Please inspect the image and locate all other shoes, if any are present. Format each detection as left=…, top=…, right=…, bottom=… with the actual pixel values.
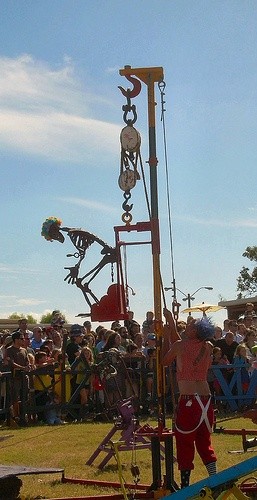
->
left=145, top=394, right=151, bottom=400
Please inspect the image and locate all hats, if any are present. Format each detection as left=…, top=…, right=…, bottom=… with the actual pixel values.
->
left=147, top=333, right=156, bottom=339
left=70, top=328, right=83, bottom=337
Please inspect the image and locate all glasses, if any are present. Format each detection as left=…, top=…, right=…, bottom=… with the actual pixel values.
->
left=18, top=338, right=25, bottom=340
left=25, top=338, right=30, bottom=340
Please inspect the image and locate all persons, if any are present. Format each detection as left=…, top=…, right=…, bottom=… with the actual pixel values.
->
left=0, top=303, right=257, bottom=426
left=160, top=308, right=218, bottom=489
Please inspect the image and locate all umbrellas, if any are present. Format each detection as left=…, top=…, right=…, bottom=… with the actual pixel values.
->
left=181, top=302, right=223, bottom=317
